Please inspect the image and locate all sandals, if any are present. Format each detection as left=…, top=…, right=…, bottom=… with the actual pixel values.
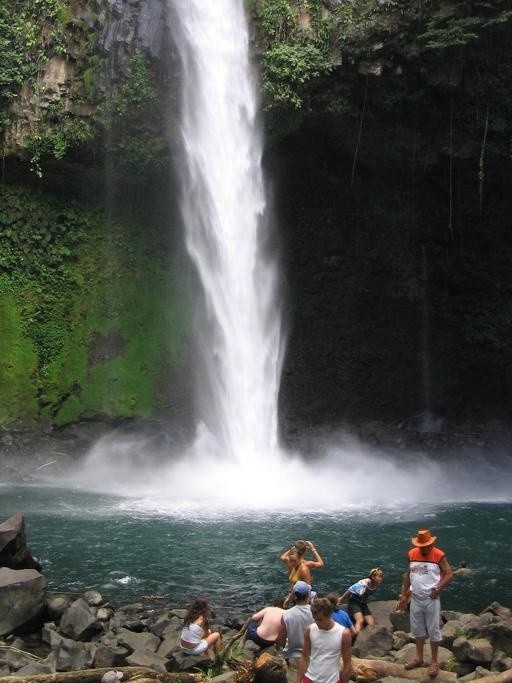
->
left=428, top=663, right=438, bottom=676
left=405, top=659, right=424, bottom=668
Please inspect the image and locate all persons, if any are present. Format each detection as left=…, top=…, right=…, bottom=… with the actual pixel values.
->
left=453, top=561, right=471, bottom=575
left=395, top=530, right=454, bottom=675
left=240, top=540, right=383, bottom=683
left=176, top=598, right=222, bottom=662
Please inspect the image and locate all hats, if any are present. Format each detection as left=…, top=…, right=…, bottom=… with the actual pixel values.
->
left=293, top=581, right=312, bottom=596
left=412, top=528, right=437, bottom=547
